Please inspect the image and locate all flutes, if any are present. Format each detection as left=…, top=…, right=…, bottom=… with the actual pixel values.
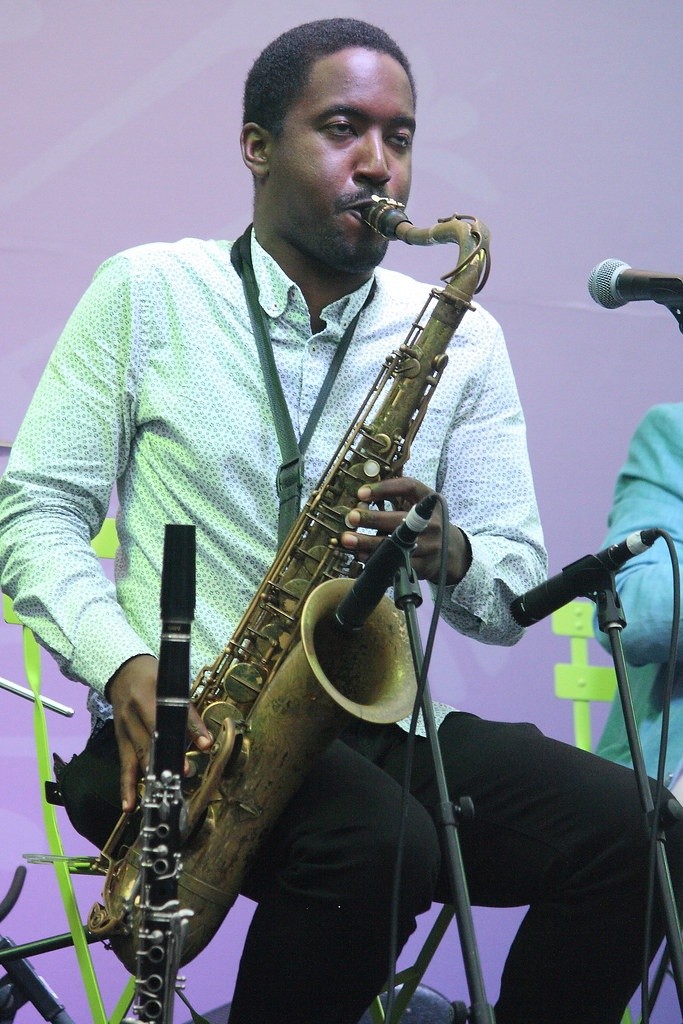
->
left=130, top=523, right=195, bottom=1024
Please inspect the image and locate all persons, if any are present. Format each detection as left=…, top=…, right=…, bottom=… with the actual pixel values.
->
left=2, top=19, right=683, bottom=1024
left=595, top=402, right=681, bottom=799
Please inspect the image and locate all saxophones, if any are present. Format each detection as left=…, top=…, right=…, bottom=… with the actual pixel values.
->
left=103, top=199, right=493, bottom=979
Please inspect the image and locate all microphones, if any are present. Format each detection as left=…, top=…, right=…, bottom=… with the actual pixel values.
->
left=509, top=528, right=663, bottom=627
left=334, top=493, right=439, bottom=632
left=587, top=259, right=683, bottom=309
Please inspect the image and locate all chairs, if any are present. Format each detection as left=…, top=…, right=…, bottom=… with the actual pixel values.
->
left=0, top=516, right=393, bottom=1024
left=548, top=599, right=621, bottom=752
